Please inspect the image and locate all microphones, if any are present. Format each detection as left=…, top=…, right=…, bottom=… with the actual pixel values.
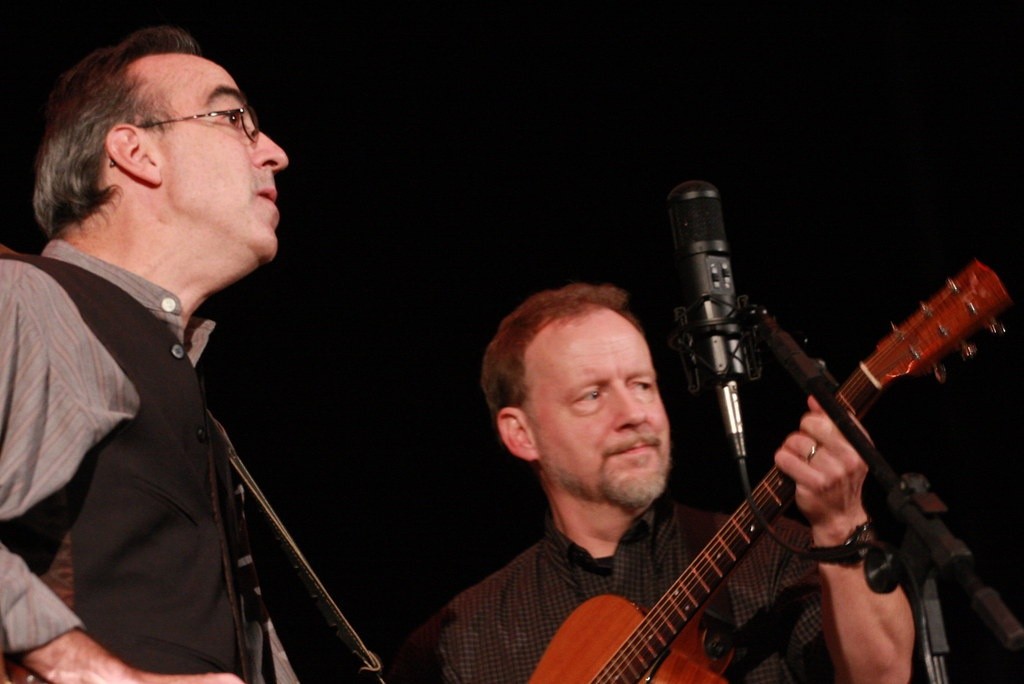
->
left=666, top=181, right=752, bottom=458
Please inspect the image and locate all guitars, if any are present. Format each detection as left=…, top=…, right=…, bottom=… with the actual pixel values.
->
left=521, top=257, right=1015, bottom=684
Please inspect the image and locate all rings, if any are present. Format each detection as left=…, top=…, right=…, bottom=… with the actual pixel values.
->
left=807, top=441, right=824, bottom=463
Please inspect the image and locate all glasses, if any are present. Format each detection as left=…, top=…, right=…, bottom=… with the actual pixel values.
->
left=109, top=102, right=261, bottom=168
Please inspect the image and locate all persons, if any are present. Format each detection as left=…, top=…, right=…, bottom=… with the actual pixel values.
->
left=388, top=282, right=914, bottom=684
left=1, top=27, right=300, bottom=683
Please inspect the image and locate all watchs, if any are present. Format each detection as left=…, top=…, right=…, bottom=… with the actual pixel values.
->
left=809, top=521, right=877, bottom=570
